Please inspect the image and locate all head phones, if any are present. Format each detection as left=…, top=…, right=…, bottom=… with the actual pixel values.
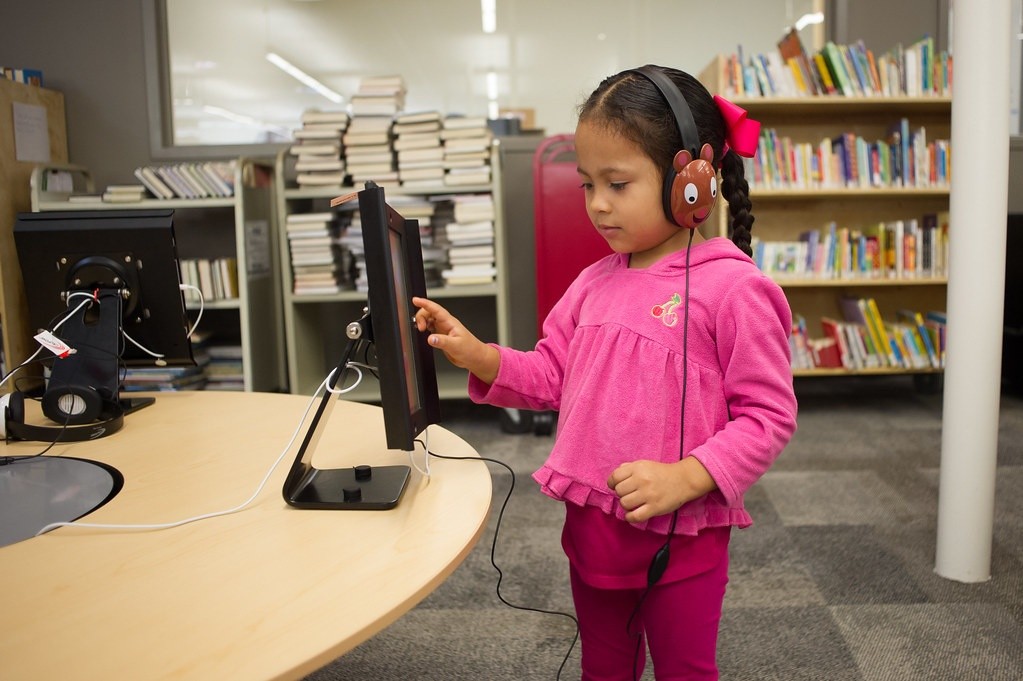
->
left=0, top=377, right=124, bottom=442
left=619, top=65, right=720, bottom=227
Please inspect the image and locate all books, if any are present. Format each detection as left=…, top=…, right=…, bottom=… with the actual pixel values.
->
left=0, top=38, right=953, bottom=393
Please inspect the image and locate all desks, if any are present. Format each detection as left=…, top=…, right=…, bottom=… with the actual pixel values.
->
left=0, top=392, right=493, bottom=680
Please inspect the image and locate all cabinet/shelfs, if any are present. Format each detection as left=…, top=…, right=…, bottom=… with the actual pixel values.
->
left=28, top=155, right=290, bottom=393
left=695, top=56, right=948, bottom=378
left=276, top=135, right=536, bottom=435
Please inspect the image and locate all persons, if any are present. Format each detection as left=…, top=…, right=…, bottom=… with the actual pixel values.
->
left=411, top=66, right=797, bottom=681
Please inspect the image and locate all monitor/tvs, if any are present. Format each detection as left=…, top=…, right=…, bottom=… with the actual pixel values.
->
left=356, top=189, right=441, bottom=450
left=14, top=208, right=198, bottom=368
